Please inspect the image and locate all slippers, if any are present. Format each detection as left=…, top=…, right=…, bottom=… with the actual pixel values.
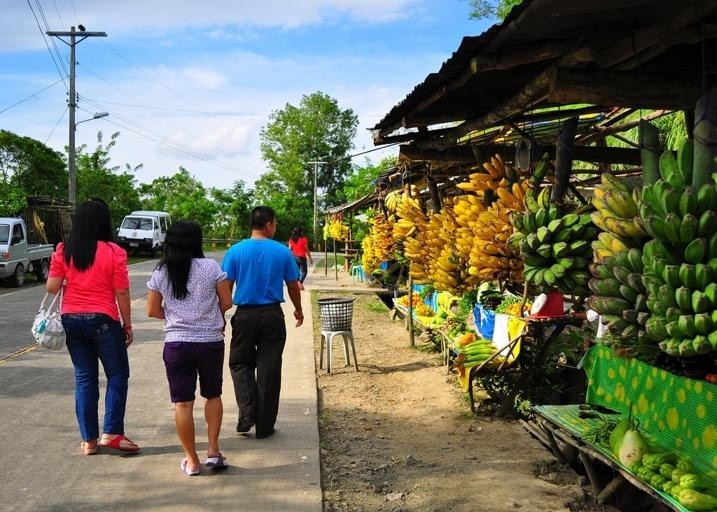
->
left=205, top=452, right=230, bottom=470
left=180, top=457, right=200, bottom=475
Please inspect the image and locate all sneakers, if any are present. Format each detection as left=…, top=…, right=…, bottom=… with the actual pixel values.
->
left=254, top=426, right=276, bottom=438
left=99, top=433, right=140, bottom=452
left=235, top=417, right=255, bottom=433
left=79, top=439, right=97, bottom=456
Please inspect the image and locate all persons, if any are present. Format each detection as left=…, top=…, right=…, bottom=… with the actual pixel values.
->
left=145, top=220, right=233, bottom=473
left=288, top=226, right=313, bottom=290
left=45, top=195, right=141, bottom=458
left=220, top=205, right=304, bottom=439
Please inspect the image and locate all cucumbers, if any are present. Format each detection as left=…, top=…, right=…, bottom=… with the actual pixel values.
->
left=630, top=452, right=717, bottom=512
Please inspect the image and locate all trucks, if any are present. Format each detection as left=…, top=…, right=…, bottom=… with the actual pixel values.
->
left=0, top=217, right=54, bottom=287
left=117, top=210, right=171, bottom=257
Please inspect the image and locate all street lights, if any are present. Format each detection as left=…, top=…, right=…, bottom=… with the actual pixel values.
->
left=75, top=112, right=109, bottom=131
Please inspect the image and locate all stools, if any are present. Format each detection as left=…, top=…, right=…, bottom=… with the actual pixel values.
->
left=318, top=331, right=359, bottom=375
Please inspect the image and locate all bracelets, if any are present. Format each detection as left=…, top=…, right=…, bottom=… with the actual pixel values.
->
left=122, top=323, right=133, bottom=331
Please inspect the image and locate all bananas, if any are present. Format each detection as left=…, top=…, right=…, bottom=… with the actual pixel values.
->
left=323, top=153, right=717, bottom=361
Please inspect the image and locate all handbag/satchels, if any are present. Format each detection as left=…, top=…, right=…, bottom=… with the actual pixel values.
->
left=30, top=307, right=67, bottom=351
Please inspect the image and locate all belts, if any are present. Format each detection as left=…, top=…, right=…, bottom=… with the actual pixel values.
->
left=62, top=312, right=106, bottom=322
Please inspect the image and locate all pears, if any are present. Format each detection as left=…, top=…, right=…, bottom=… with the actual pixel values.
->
left=608, top=403, right=648, bottom=468
